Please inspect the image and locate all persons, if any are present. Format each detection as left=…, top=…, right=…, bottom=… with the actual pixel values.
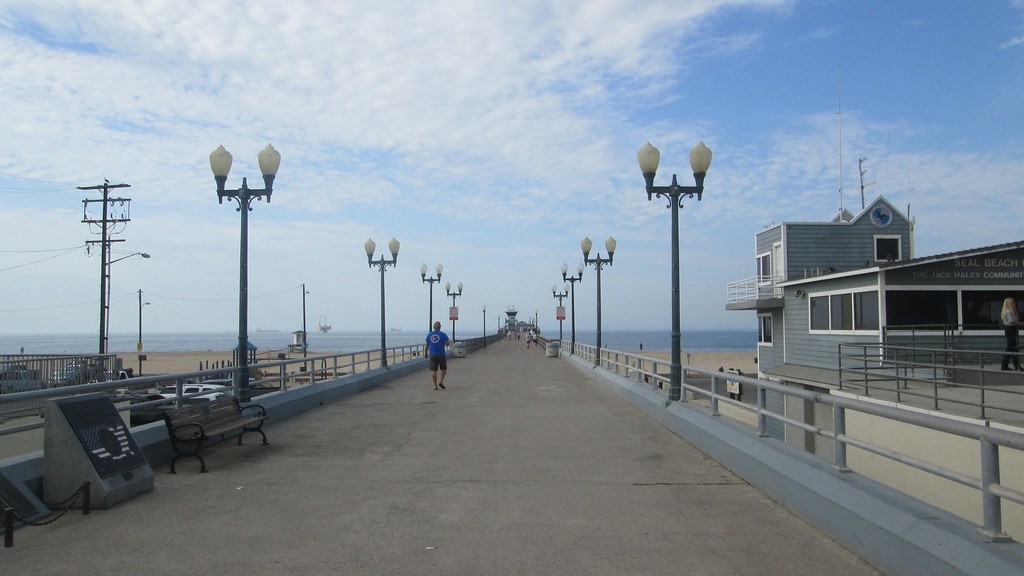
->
left=498, top=328, right=538, bottom=349
left=425, top=321, right=450, bottom=390
left=1001, top=298, right=1024, bottom=371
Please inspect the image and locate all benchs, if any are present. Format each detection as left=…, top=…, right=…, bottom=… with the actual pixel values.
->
left=160, top=395, right=269, bottom=474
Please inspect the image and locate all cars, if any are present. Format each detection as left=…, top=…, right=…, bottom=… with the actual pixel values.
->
left=108, top=376, right=252, bottom=408
left=1, top=357, right=98, bottom=397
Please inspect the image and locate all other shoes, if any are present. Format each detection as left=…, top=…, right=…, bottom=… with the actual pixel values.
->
left=434, top=387, right=438, bottom=390
left=439, top=382, right=446, bottom=389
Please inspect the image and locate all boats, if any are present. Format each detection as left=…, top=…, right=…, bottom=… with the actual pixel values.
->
left=316, top=323, right=331, bottom=331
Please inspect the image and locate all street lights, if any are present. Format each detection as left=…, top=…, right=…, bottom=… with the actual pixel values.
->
left=445, top=281, right=463, bottom=349
left=637, top=140, right=715, bottom=405
left=580, top=234, right=616, bottom=365
left=561, top=261, right=585, bottom=356
left=209, top=142, right=281, bottom=403
left=421, top=262, right=444, bottom=334
left=100, top=252, right=151, bottom=359
left=552, top=284, right=570, bottom=357
left=482, top=304, right=486, bottom=348
left=364, top=237, right=400, bottom=369
left=534, top=309, right=539, bottom=340
